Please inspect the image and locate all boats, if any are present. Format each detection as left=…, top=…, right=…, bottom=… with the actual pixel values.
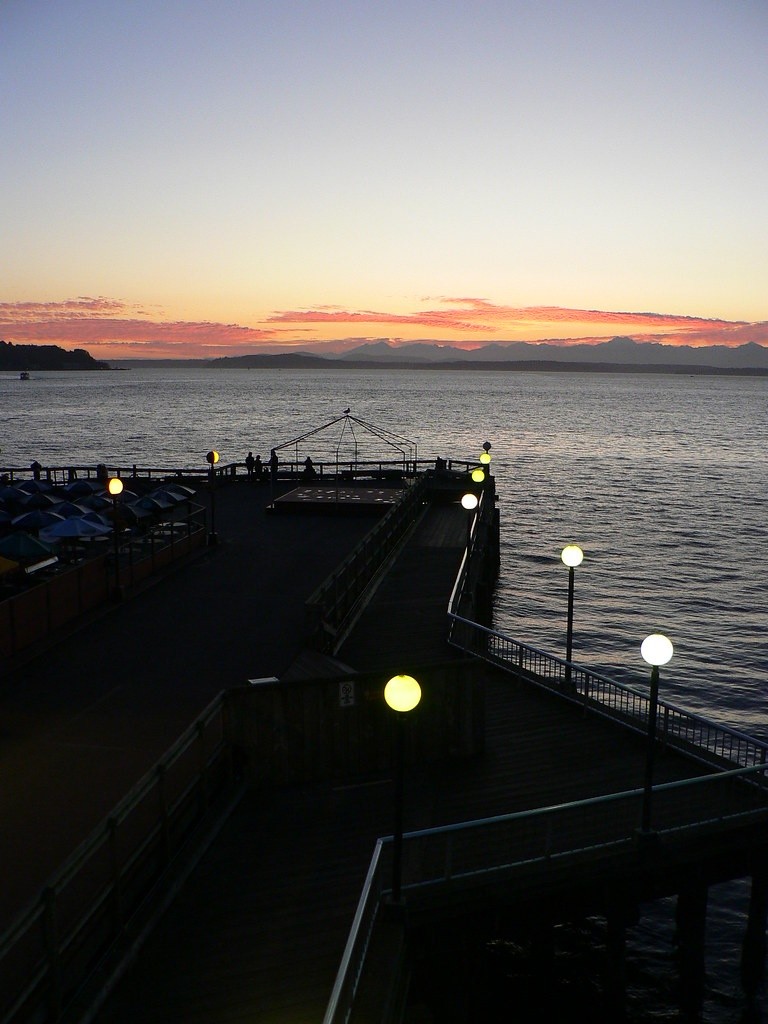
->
left=19, top=371, right=31, bottom=382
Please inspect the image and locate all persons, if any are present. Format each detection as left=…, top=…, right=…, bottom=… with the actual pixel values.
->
left=268, top=450, right=278, bottom=484
left=304, top=457, right=313, bottom=469
left=245, top=452, right=263, bottom=481
left=435, top=457, right=443, bottom=473
